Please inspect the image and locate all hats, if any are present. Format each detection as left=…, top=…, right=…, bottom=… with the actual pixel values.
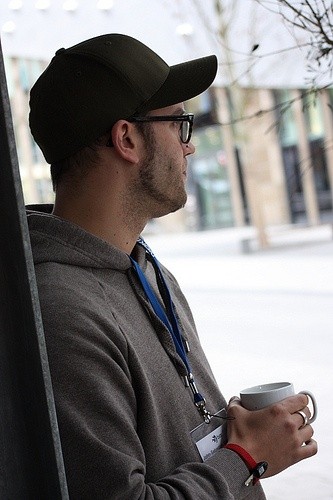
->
left=29, top=33, right=219, bottom=165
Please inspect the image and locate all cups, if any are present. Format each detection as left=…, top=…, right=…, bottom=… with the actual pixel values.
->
left=239, top=382, right=318, bottom=425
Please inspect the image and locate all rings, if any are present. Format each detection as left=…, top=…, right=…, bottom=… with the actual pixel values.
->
left=295, top=411, right=307, bottom=428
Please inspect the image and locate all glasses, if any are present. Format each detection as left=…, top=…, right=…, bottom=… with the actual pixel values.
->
left=130, top=112, right=194, bottom=143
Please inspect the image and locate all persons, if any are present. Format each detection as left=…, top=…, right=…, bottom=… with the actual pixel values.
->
left=23, top=33, right=317, bottom=500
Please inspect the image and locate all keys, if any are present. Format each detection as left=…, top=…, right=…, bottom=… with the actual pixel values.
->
left=244, top=462, right=268, bottom=486
